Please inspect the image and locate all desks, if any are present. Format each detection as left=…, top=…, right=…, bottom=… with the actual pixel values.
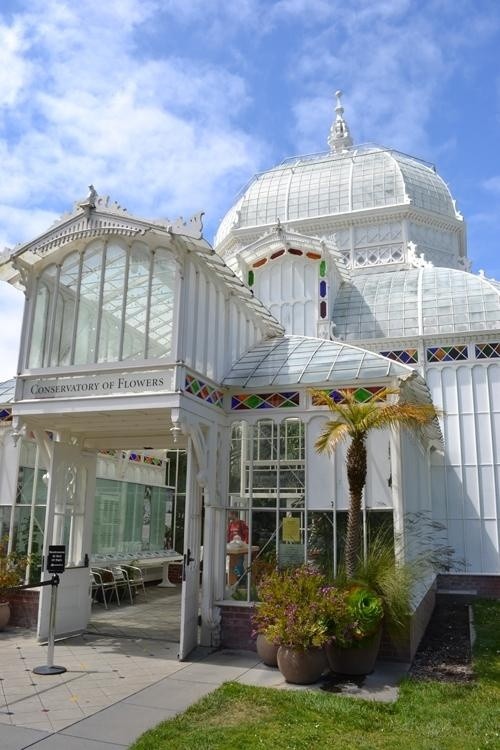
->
left=225, top=542, right=259, bottom=586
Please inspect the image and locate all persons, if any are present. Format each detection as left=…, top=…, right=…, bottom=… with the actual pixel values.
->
left=225, top=509, right=248, bottom=583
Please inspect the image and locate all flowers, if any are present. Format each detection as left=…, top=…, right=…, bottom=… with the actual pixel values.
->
left=249, top=563, right=358, bottom=659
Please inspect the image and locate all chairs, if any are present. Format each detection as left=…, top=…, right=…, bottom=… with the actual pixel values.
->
left=89, top=567, right=121, bottom=610
left=109, top=563, right=148, bottom=606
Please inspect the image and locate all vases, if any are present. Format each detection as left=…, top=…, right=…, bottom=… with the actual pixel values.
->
left=256, top=634, right=279, bottom=668
left=277, top=644, right=328, bottom=685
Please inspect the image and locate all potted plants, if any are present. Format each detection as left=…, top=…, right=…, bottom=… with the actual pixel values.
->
left=326, top=507, right=464, bottom=674
left=0, top=534, right=37, bottom=632
